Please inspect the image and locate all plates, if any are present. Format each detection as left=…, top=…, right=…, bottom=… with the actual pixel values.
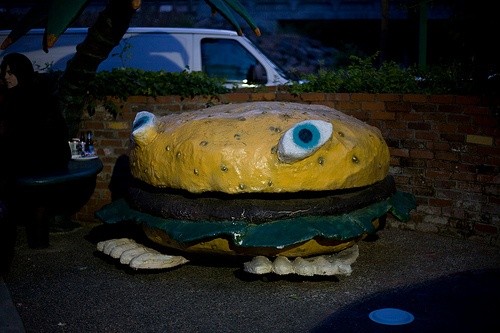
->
left=71, top=155, right=98, bottom=161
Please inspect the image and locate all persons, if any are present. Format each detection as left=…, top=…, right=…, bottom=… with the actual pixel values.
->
left=1, top=49, right=72, bottom=265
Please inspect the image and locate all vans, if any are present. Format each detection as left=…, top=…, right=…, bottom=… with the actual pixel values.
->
left=0, top=28, right=308, bottom=87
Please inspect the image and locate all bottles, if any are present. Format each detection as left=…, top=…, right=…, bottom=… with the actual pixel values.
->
left=79, top=130, right=88, bottom=157
left=85, top=131, right=94, bottom=157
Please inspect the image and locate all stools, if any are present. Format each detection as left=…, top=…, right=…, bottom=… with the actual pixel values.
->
left=10, top=148, right=104, bottom=249
